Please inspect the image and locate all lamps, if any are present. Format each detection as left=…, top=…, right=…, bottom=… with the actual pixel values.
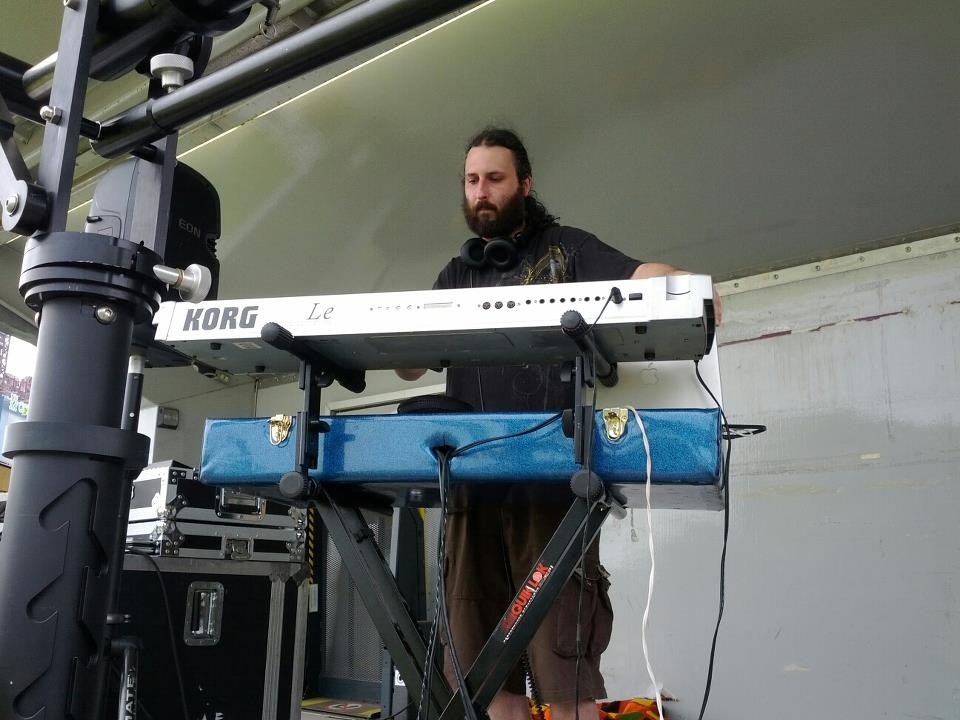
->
left=157, top=406, right=179, bottom=430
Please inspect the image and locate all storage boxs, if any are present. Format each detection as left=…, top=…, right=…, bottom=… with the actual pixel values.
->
left=107, top=458, right=311, bottom=720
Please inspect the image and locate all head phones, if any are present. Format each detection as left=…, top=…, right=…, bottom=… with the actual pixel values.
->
left=460, top=223, right=536, bottom=271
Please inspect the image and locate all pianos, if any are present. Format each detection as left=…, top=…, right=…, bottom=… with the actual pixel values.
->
left=150, top=274, right=724, bottom=720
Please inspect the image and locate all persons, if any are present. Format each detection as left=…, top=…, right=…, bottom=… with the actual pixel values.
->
left=395, top=129, right=723, bottom=720
